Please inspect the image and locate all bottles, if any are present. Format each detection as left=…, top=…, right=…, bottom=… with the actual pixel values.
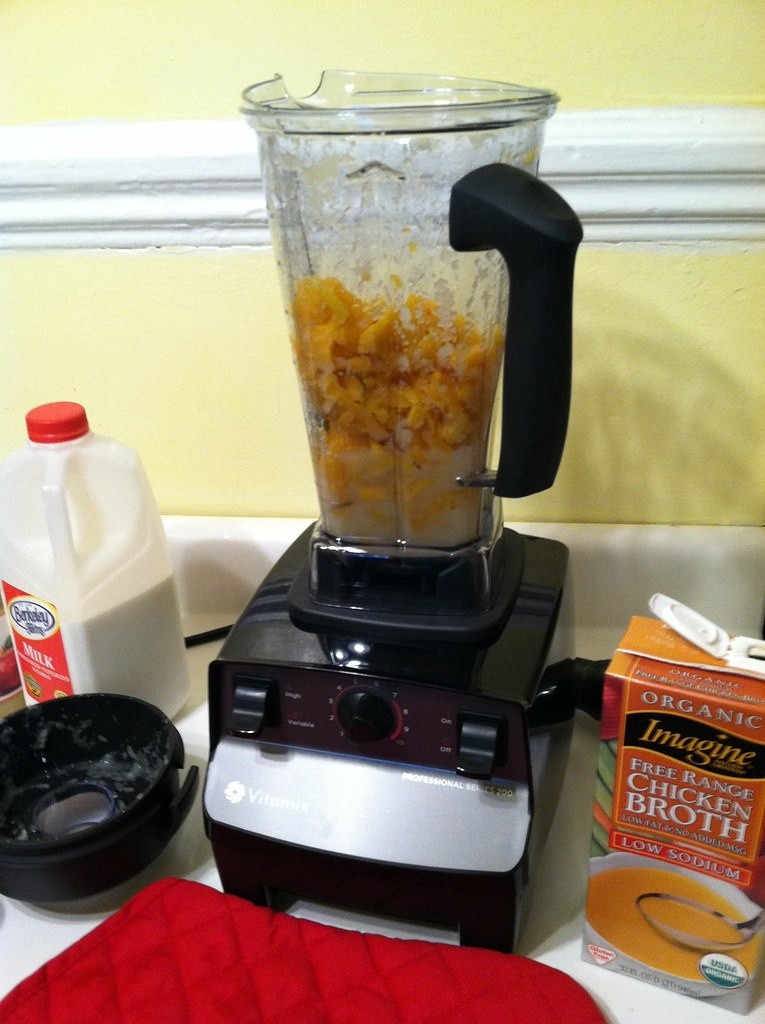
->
left=0, top=401, right=193, bottom=718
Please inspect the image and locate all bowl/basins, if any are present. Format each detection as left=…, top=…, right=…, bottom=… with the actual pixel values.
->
left=0, top=693, right=202, bottom=904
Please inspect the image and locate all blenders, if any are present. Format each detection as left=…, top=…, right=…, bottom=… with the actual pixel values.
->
left=198, top=68, right=586, bottom=956
